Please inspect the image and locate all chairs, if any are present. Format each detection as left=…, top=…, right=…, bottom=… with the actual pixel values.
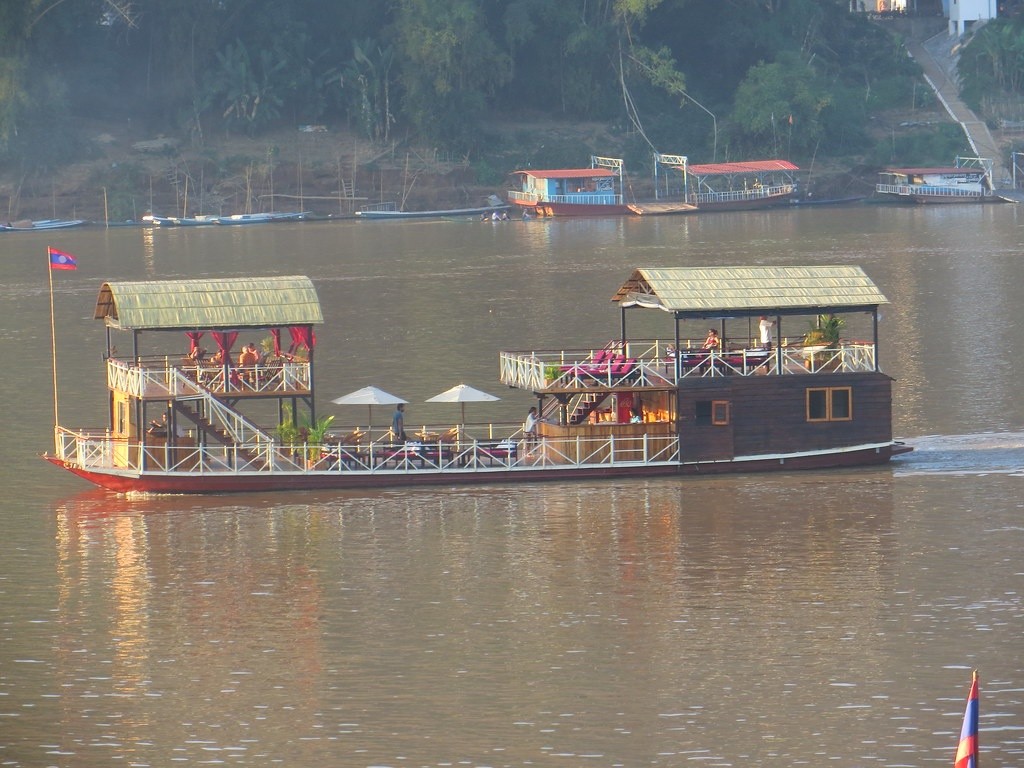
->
left=568, top=355, right=626, bottom=387
left=561, top=353, right=617, bottom=384
left=558, top=350, right=606, bottom=384
left=182, top=353, right=282, bottom=390
left=577, top=360, right=635, bottom=388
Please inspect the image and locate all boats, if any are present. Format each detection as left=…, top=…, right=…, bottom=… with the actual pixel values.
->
left=354, top=205, right=511, bottom=220
left=38, top=267, right=917, bottom=494
left=505, top=169, right=630, bottom=217
left=669, top=160, right=800, bottom=212
left=144, top=211, right=312, bottom=226
left=0, top=218, right=88, bottom=232
left=875, top=167, right=988, bottom=204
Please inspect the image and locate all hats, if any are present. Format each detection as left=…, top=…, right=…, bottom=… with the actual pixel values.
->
left=760, top=314, right=769, bottom=320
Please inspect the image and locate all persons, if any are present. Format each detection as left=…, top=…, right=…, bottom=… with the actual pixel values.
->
left=702, top=329, right=720, bottom=349
left=393, top=403, right=410, bottom=441
left=479, top=208, right=510, bottom=221
left=239, top=343, right=260, bottom=370
left=629, top=408, right=642, bottom=423
left=825, top=313, right=839, bottom=361
left=190, top=344, right=235, bottom=380
left=759, top=316, right=782, bottom=369
left=525, top=407, right=542, bottom=450
left=152, top=413, right=168, bottom=428
left=523, top=209, right=528, bottom=217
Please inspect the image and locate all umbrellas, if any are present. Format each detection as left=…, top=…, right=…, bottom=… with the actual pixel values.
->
left=330, top=384, right=409, bottom=441
left=423, top=383, right=501, bottom=447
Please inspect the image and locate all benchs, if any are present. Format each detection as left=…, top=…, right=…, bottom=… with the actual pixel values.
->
left=476, top=439, right=519, bottom=468
left=662, top=345, right=770, bottom=374
left=383, top=441, right=453, bottom=468
left=319, top=441, right=357, bottom=468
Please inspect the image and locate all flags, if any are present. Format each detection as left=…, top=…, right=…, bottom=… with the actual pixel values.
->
left=45, top=247, right=78, bottom=271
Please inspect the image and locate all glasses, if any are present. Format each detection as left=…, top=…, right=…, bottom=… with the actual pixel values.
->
left=709, top=332, right=712, bottom=333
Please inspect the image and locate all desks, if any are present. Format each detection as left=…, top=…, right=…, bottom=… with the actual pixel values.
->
left=359, top=452, right=387, bottom=468
left=450, top=450, right=478, bottom=467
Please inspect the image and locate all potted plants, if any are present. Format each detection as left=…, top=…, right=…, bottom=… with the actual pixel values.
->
left=541, top=359, right=567, bottom=388
left=299, top=409, right=335, bottom=471
left=271, top=405, right=309, bottom=463
left=799, top=331, right=823, bottom=370
